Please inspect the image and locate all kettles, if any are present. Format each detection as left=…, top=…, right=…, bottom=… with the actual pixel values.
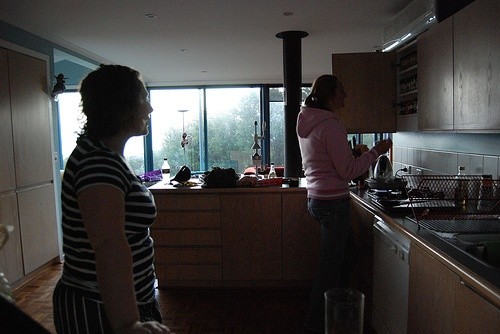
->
left=373, top=155, right=393, bottom=180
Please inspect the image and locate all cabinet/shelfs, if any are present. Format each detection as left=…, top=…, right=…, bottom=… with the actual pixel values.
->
left=417, top=0, right=500, bottom=134
left=332, top=38, right=417, bottom=133
left=140, top=173, right=500, bottom=334
left=0, top=47, right=59, bottom=284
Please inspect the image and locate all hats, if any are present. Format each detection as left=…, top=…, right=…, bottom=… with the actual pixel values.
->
left=171, top=166, right=190, bottom=182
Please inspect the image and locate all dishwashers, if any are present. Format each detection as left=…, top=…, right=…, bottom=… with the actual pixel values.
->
left=370, top=214, right=412, bottom=334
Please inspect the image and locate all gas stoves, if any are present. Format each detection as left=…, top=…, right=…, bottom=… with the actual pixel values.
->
left=350, top=186, right=456, bottom=213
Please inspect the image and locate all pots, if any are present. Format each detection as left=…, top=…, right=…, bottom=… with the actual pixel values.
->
left=365, top=180, right=407, bottom=189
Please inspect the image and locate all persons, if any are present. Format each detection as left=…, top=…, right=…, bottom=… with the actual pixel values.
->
left=296, top=73, right=391, bottom=328
left=53, top=64, right=173, bottom=334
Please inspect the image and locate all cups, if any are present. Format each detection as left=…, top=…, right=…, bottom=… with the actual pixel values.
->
left=288, top=178, right=299, bottom=187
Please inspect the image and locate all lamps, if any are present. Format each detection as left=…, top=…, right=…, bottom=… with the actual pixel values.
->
left=51, top=73, right=66, bottom=95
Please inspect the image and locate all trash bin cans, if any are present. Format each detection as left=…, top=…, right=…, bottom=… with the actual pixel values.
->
left=324, top=287, right=365, bottom=334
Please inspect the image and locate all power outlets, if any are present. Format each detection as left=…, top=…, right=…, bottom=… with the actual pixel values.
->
left=403, top=166, right=411, bottom=174
left=416, top=169, right=423, bottom=178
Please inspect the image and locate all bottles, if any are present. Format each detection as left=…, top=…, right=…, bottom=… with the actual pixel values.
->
left=400, top=73, right=416, bottom=93
left=268, top=162, right=277, bottom=178
left=161, top=157, right=170, bottom=184
left=400, top=50, right=417, bottom=71
left=455, top=166, right=494, bottom=206
left=401, top=96, right=417, bottom=115
